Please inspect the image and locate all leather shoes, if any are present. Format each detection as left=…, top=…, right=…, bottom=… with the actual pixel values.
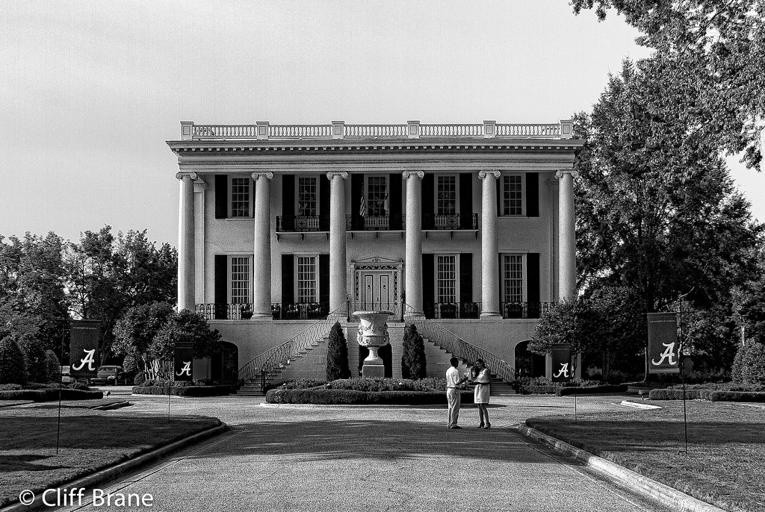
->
left=447, top=425, right=462, bottom=429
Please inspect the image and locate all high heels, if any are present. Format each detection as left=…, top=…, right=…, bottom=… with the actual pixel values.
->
left=476, top=422, right=490, bottom=429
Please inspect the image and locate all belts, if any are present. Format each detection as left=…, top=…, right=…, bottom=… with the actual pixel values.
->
left=449, top=387, right=460, bottom=390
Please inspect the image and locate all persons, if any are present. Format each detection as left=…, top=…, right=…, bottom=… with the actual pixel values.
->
left=468, top=359, right=490, bottom=429
left=445, top=358, right=469, bottom=430
